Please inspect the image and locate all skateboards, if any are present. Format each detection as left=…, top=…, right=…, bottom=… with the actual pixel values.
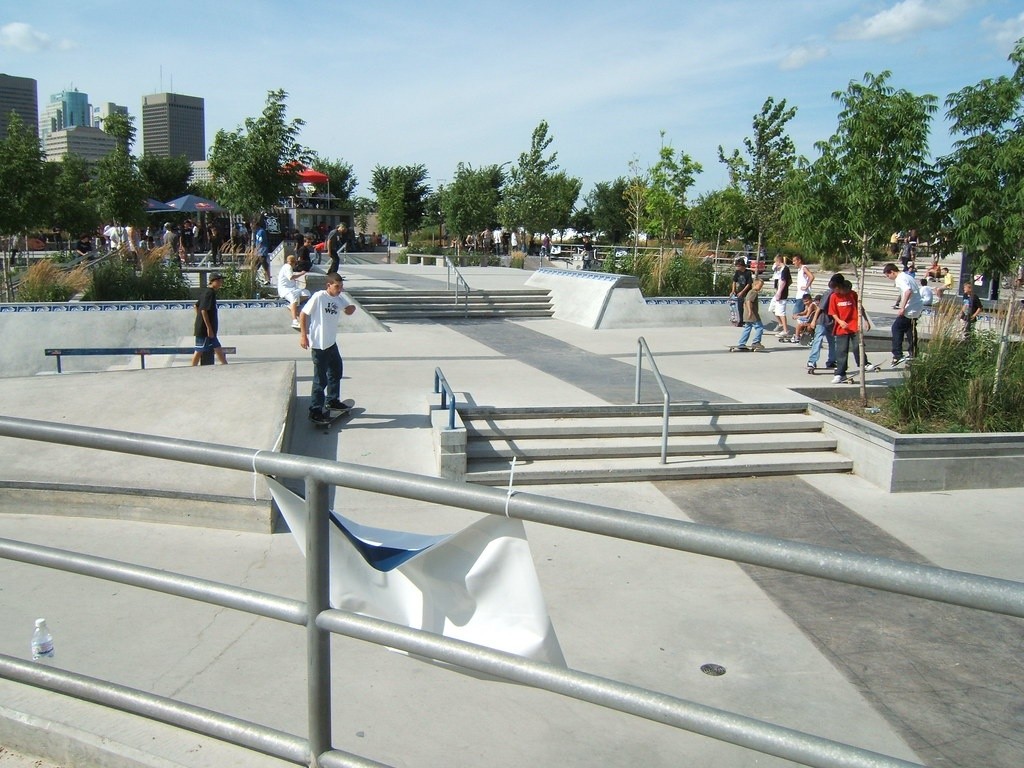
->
left=341, top=277, right=346, bottom=280
left=725, top=345, right=763, bottom=352
left=804, top=368, right=837, bottom=374
left=778, top=336, right=792, bottom=343
left=892, top=356, right=910, bottom=368
left=308, top=398, right=355, bottom=426
left=727, top=294, right=741, bottom=327
left=891, top=306, right=899, bottom=309
left=840, top=359, right=887, bottom=384
left=800, top=310, right=815, bottom=346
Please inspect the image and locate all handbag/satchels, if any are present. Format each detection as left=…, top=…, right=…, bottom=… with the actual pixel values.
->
left=179, top=248, right=185, bottom=259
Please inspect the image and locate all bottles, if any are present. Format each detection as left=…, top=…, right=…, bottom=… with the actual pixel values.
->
left=32, top=618, right=54, bottom=666
left=857, top=407, right=881, bottom=414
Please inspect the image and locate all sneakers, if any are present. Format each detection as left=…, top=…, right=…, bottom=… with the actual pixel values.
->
left=832, top=374, right=847, bottom=383
left=775, top=330, right=789, bottom=337
left=308, top=408, right=324, bottom=423
left=327, top=400, right=352, bottom=410
left=857, top=364, right=874, bottom=370
left=292, top=323, right=300, bottom=328
left=774, top=324, right=783, bottom=332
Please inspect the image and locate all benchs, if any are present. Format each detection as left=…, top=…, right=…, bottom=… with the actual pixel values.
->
left=447, top=255, right=488, bottom=267
left=406, top=254, right=448, bottom=267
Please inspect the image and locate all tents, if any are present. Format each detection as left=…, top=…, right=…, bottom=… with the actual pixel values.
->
left=277, top=161, right=329, bottom=209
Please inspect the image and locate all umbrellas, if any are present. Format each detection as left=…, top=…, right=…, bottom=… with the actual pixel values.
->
left=144, top=200, right=179, bottom=212
left=167, top=194, right=228, bottom=212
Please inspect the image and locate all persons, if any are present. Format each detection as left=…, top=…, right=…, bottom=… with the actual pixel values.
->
left=580, top=237, right=593, bottom=269
left=730, top=229, right=982, bottom=385
left=706, top=253, right=715, bottom=262
left=191, top=272, right=227, bottom=365
left=300, top=272, right=356, bottom=423
left=466, top=227, right=510, bottom=255
left=138, top=218, right=200, bottom=266
left=528, top=234, right=549, bottom=255
left=104, top=219, right=139, bottom=252
left=77, top=233, right=92, bottom=259
left=356, top=232, right=379, bottom=246
left=327, top=222, right=347, bottom=274
left=239, top=224, right=248, bottom=245
left=303, top=221, right=329, bottom=243
left=297, top=237, right=325, bottom=273
left=281, top=182, right=336, bottom=209
left=278, top=255, right=310, bottom=328
left=207, top=221, right=226, bottom=267
left=511, top=230, right=517, bottom=251
left=249, top=221, right=271, bottom=285
left=311, top=241, right=325, bottom=264
left=293, top=230, right=304, bottom=257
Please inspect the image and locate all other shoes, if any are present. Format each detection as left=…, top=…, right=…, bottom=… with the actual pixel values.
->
left=891, top=356, right=905, bottom=366
left=752, top=343, right=765, bottom=350
left=807, top=361, right=817, bottom=367
left=738, top=346, right=749, bottom=350
left=906, top=355, right=919, bottom=360
left=827, top=363, right=837, bottom=369
left=790, top=336, right=800, bottom=341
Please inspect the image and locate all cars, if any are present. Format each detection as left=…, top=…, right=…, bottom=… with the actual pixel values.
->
left=358, top=233, right=387, bottom=246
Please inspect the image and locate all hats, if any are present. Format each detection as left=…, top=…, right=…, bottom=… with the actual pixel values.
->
left=209, top=272, right=227, bottom=282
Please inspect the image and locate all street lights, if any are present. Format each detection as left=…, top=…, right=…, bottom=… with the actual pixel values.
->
left=437, top=211, right=443, bottom=247
